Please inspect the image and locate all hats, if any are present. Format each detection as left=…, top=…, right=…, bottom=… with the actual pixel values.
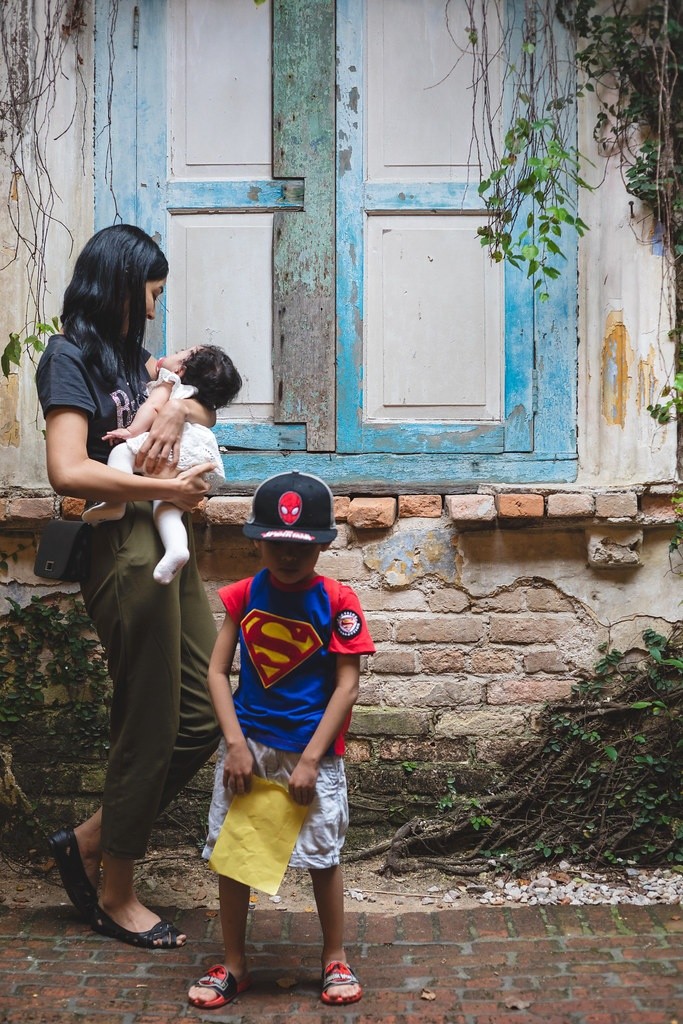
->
left=239, top=470, right=339, bottom=544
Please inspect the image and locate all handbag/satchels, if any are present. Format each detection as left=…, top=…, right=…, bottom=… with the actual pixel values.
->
left=34, top=518, right=94, bottom=583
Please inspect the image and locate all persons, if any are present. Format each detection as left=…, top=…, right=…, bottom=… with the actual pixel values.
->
left=36, top=225, right=225, bottom=947
left=187, top=471, right=376, bottom=1009
left=80, top=342, right=243, bottom=585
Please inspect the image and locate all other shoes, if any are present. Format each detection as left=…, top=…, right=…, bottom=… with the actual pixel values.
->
left=90, top=904, right=188, bottom=950
left=49, top=824, right=99, bottom=924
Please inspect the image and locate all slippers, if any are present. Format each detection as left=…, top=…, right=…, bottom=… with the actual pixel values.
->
left=320, top=960, right=363, bottom=1006
left=185, top=962, right=253, bottom=1009
